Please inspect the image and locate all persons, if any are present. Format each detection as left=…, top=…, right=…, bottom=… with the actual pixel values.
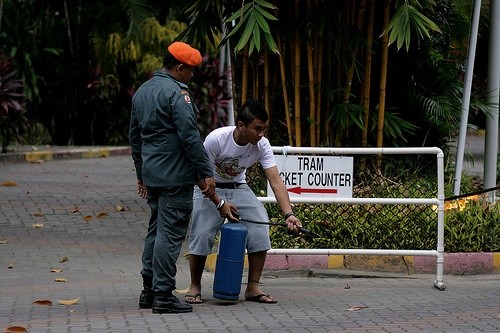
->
left=184, top=101, right=303, bottom=304
left=129, top=42, right=216, bottom=313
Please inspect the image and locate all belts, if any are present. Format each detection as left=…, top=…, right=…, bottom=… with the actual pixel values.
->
left=216, top=182, right=244, bottom=189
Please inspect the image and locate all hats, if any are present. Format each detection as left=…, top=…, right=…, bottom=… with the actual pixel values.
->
left=169, top=42, right=202, bottom=67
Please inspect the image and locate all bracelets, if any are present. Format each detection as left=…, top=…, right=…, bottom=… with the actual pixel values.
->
left=217, top=198, right=225, bottom=210
left=284, top=212, right=296, bottom=221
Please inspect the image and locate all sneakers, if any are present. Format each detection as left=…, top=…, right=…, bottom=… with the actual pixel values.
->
left=152, top=291, right=193, bottom=313
left=139, top=289, right=153, bottom=309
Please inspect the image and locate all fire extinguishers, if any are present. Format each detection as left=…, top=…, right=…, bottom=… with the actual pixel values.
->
left=212, top=209, right=249, bottom=300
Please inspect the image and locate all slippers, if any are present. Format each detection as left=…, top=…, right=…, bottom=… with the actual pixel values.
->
left=184, top=293, right=203, bottom=304
left=245, top=293, right=277, bottom=303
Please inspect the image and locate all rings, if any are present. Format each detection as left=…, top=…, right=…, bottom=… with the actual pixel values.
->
left=141, top=189, right=142, bottom=190
left=143, top=190, right=145, bottom=191
